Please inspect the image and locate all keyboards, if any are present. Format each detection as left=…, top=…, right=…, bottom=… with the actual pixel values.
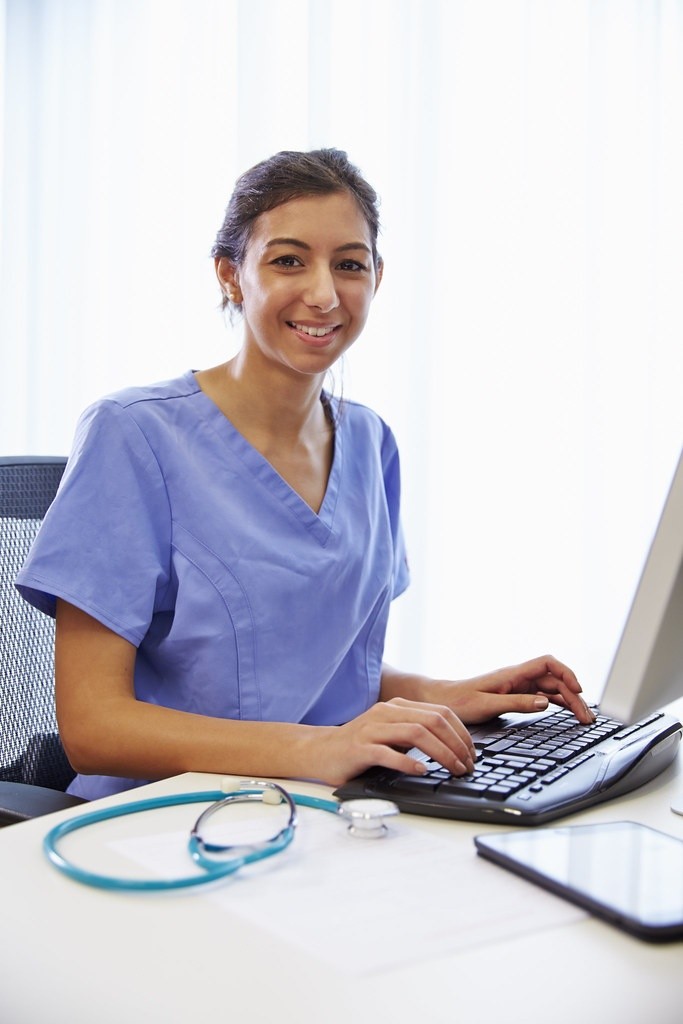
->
left=333, top=703, right=683, bottom=827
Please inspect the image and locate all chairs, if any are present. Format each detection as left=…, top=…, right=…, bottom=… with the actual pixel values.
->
left=1, top=456, right=89, bottom=829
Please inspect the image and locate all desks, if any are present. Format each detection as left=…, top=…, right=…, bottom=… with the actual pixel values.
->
left=2, top=772, right=683, bottom=1024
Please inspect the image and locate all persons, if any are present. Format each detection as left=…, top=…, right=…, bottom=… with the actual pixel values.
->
left=14, top=148, right=594, bottom=802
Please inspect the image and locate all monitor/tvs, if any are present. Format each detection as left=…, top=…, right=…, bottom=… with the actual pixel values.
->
left=599, top=451, right=683, bottom=726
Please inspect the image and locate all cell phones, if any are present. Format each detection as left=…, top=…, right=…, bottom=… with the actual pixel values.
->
left=474, top=820, right=683, bottom=938
